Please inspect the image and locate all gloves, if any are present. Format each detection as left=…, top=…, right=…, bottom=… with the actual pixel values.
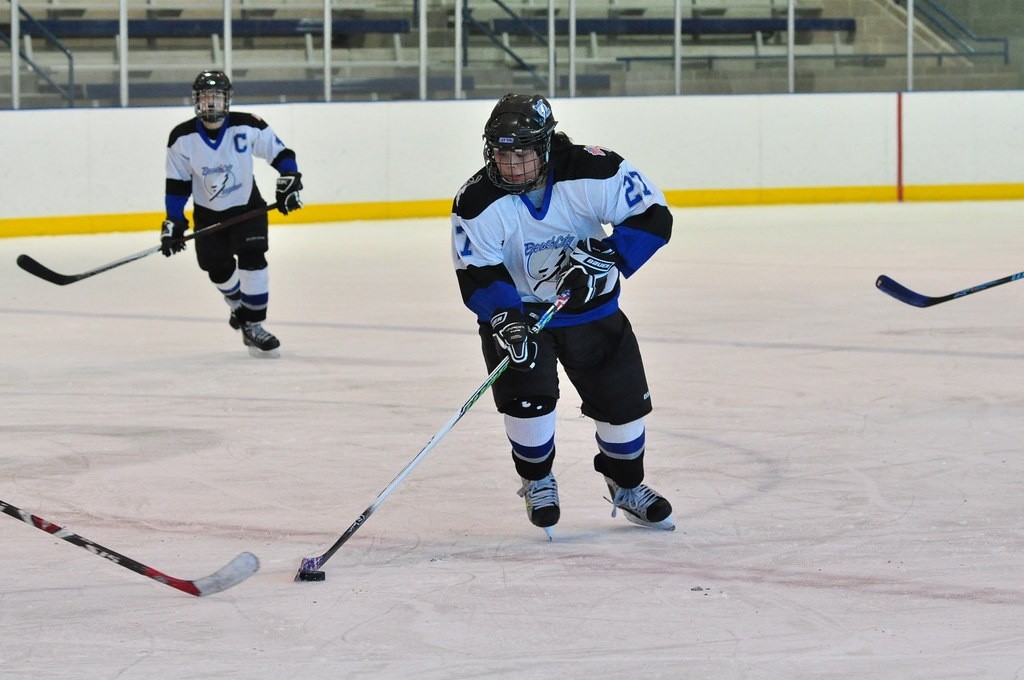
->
left=275, top=173, right=303, bottom=215
left=489, top=308, right=539, bottom=372
left=556, top=237, right=618, bottom=312
left=161, top=219, right=190, bottom=256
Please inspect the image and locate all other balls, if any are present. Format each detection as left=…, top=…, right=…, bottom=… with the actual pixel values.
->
left=300, top=570, right=325, bottom=580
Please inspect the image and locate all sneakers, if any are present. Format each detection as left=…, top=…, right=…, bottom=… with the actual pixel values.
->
left=224, top=290, right=243, bottom=329
left=242, top=319, right=280, bottom=358
left=516, top=471, right=560, bottom=542
left=602, top=476, right=676, bottom=531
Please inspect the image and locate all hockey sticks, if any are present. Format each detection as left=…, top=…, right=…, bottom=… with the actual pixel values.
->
left=1, top=500, right=260, bottom=598
left=294, top=290, right=570, bottom=580
left=16, top=200, right=280, bottom=286
left=876, top=272, right=1024, bottom=309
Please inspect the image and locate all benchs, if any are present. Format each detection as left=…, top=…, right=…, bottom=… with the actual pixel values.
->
left=0, top=0, right=857, bottom=110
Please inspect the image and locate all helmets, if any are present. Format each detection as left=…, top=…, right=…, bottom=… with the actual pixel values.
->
left=484, top=93, right=559, bottom=193
left=192, top=71, right=232, bottom=122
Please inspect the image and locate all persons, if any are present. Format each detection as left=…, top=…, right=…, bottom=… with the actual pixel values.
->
left=450, top=92, right=674, bottom=541
left=159, top=69, right=304, bottom=353
